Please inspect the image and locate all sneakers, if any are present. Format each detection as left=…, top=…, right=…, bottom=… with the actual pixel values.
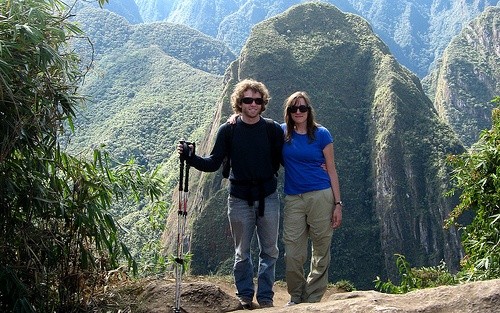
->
left=236, top=296, right=252, bottom=310
left=260, top=300, right=274, bottom=308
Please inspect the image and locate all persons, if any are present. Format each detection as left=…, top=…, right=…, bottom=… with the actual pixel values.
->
left=225, top=90, right=343, bottom=309
left=177, top=78, right=329, bottom=309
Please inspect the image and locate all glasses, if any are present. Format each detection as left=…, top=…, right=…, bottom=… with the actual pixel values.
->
left=242, top=97, right=264, bottom=104
left=289, top=105, right=308, bottom=112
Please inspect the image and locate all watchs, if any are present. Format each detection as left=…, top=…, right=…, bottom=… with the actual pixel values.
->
left=335, top=201, right=343, bottom=207
